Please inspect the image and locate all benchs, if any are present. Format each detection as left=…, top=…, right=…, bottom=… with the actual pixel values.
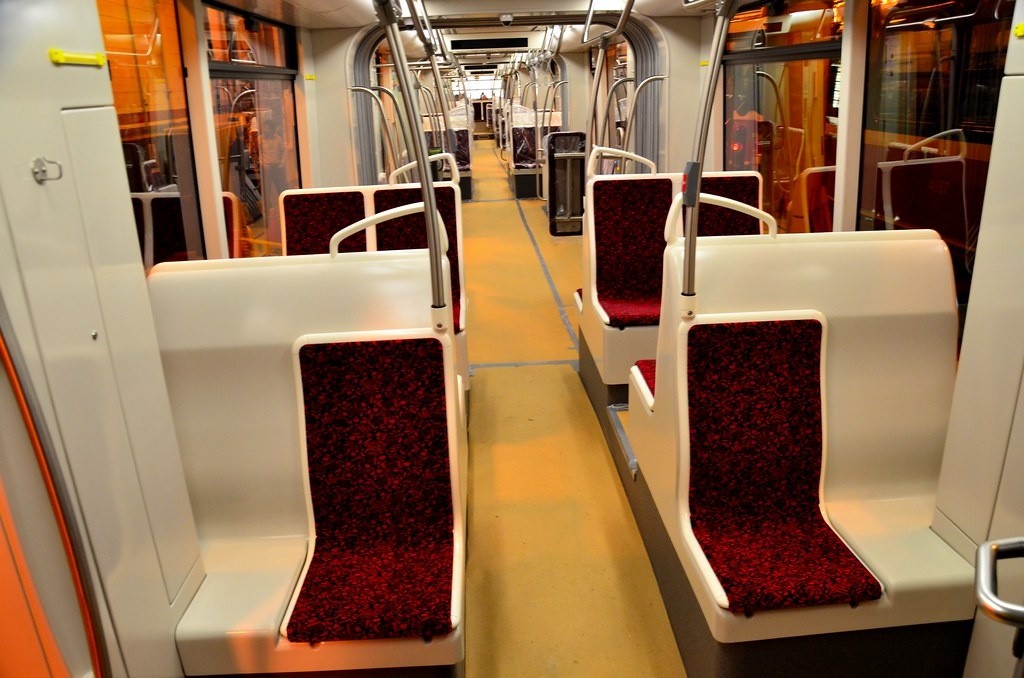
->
left=148, top=102, right=976, bottom=675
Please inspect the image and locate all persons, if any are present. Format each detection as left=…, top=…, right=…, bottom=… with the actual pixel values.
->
left=478, top=95, right=488, bottom=100
left=226, top=106, right=294, bottom=225
